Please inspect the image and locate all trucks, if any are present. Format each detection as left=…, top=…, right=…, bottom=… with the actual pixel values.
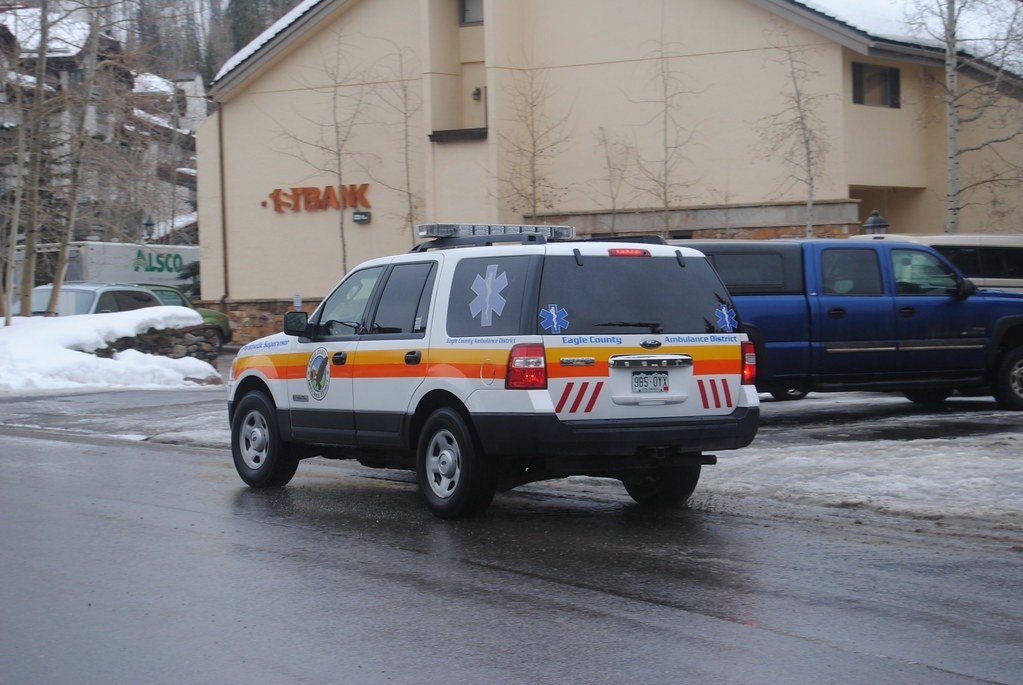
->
left=10, top=239, right=201, bottom=308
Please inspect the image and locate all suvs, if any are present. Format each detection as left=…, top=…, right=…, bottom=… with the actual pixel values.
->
left=227, top=225, right=761, bottom=523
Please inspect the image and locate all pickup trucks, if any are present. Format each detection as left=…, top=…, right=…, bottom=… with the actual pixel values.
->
left=660, top=237, right=1023, bottom=415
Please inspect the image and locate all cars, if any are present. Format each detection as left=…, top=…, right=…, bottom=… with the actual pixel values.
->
left=121, top=282, right=234, bottom=357
left=0, top=281, right=164, bottom=318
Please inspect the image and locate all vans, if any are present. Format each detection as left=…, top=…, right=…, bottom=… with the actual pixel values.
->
left=825, top=234, right=1023, bottom=296
